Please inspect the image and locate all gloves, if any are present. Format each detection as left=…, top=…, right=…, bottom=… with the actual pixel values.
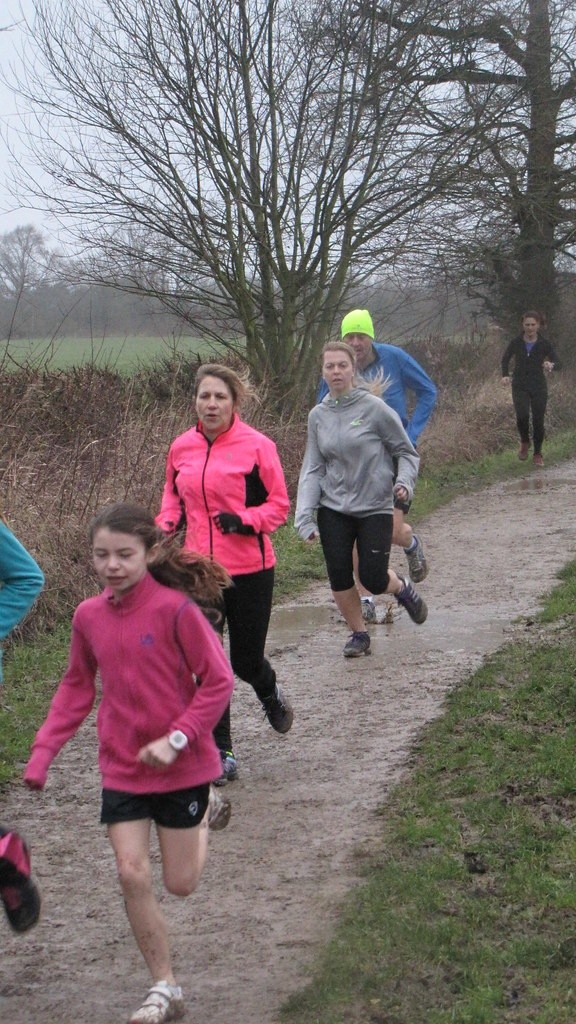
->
left=214, top=513, right=242, bottom=534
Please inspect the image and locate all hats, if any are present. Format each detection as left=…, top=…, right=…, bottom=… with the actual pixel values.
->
left=341, top=308, right=375, bottom=340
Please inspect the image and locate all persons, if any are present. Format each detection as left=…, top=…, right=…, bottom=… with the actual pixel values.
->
left=155, top=363, right=291, bottom=785
left=295, top=341, right=429, bottom=656
left=500, top=310, right=557, bottom=468
left=23, top=502, right=234, bottom=1023
left=1, top=517, right=45, bottom=931
left=317, top=310, right=435, bottom=625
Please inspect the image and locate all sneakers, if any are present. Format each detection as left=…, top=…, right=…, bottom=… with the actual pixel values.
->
left=533, top=451, right=544, bottom=466
left=260, top=683, right=294, bottom=733
left=0, top=828, right=41, bottom=931
left=394, top=577, right=429, bottom=624
left=341, top=632, right=373, bottom=657
left=212, top=750, right=239, bottom=784
left=337, top=599, right=377, bottom=623
left=403, top=533, right=428, bottom=582
left=128, top=982, right=186, bottom=1024
left=518, top=443, right=531, bottom=461
left=207, top=782, right=231, bottom=831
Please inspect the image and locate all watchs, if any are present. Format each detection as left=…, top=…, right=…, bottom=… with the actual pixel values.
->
left=166, top=729, right=187, bottom=751
